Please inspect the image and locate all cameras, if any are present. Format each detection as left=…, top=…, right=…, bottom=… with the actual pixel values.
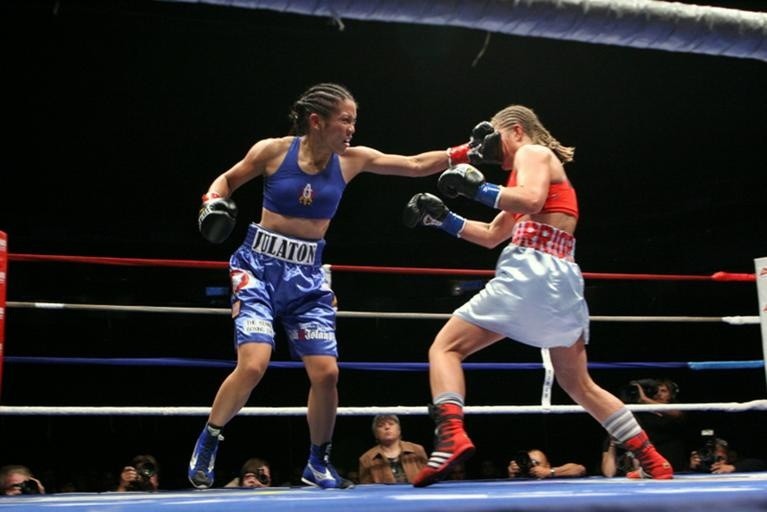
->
left=513, top=451, right=537, bottom=479
left=695, top=448, right=718, bottom=470
left=15, top=479, right=41, bottom=495
left=130, top=460, right=155, bottom=489
left=254, top=468, right=269, bottom=485
left=616, top=455, right=635, bottom=472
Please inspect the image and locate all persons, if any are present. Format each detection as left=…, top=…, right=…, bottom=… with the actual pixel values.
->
left=601, top=436, right=640, bottom=476
left=225, top=460, right=271, bottom=488
left=689, top=438, right=737, bottom=473
left=1, top=465, right=47, bottom=496
left=635, top=379, right=688, bottom=471
left=407, top=104, right=674, bottom=489
left=509, top=450, right=586, bottom=478
left=338, top=465, right=359, bottom=483
left=119, top=456, right=159, bottom=491
left=359, top=413, right=426, bottom=484
left=185, top=82, right=503, bottom=491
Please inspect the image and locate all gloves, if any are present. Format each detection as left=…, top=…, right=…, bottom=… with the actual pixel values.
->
left=447, top=119, right=502, bottom=169
left=436, top=163, right=504, bottom=211
left=195, top=191, right=239, bottom=244
left=406, top=190, right=466, bottom=239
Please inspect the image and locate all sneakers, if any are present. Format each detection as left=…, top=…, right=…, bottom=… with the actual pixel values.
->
left=622, top=430, right=674, bottom=482
left=411, top=401, right=475, bottom=487
left=186, top=423, right=225, bottom=490
left=298, top=442, right=357, bottom=491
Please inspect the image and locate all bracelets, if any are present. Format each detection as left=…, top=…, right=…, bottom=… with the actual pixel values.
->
left=550, top=468, right=554, bottom=477
